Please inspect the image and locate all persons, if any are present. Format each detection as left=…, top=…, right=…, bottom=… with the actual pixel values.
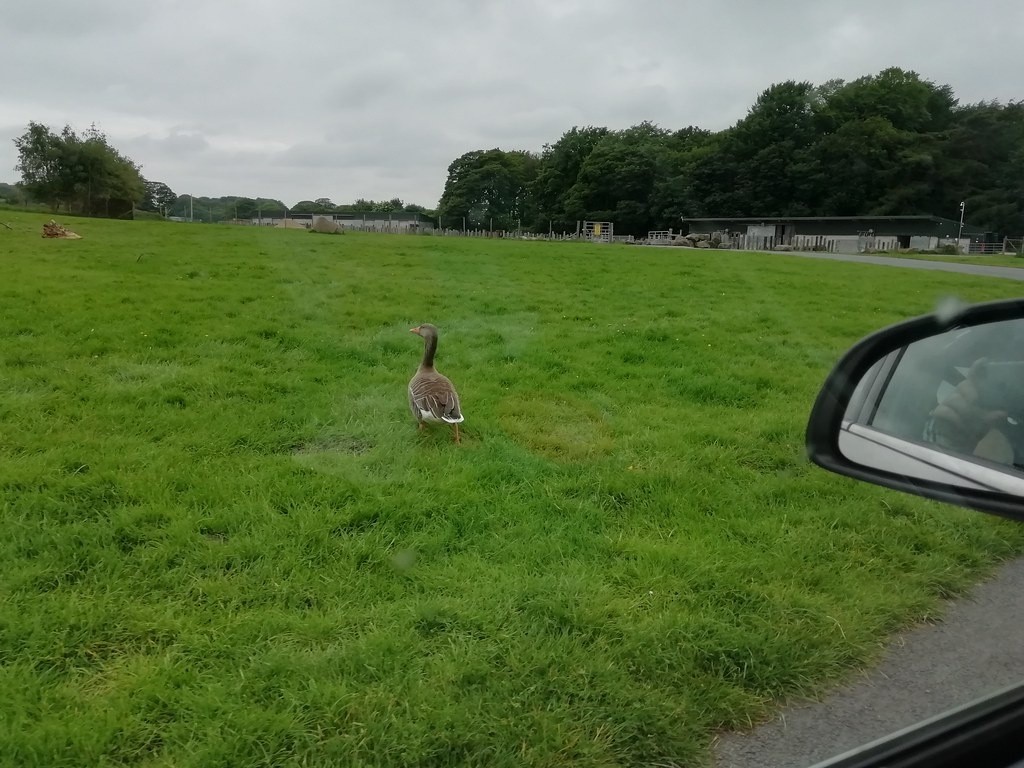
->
left=979, top=240, right=986, bottom=253
left=925, top=330, right=1024, bottom=473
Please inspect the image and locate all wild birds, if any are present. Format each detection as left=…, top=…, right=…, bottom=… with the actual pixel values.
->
left=406, top=322, right=464, bottom=445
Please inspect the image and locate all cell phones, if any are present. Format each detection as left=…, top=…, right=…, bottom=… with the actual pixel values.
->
left=982, top=361, right=1024, bottom=413
